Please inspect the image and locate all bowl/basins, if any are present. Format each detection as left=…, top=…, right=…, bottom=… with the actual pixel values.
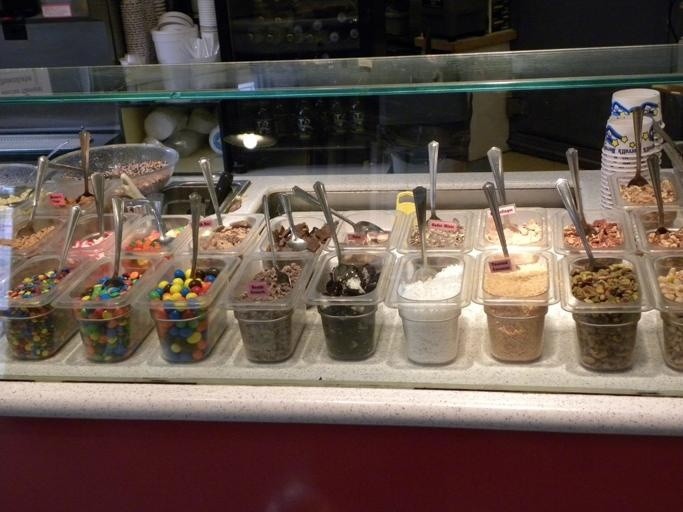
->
left=45, top=143, right=181, bottom=195
left=0, top=163, right=40, bottom=211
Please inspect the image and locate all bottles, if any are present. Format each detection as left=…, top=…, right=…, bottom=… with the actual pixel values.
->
left=243, top=9, right=358, bottom=46
left=251, top=97, right=368, bottom=140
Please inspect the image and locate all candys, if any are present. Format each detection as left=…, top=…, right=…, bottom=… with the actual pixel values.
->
left=0, top=230, right=221, bottom=363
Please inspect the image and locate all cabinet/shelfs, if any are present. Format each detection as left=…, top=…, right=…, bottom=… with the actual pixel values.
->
left=0, top=42, right=682, bottom=510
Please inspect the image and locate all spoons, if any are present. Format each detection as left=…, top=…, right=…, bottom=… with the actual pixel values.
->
left=646, top=154, right=671, bottom=238
left=263, top=194, right=290, bottom=286
left=554, top=178, right=610, bottom=273
left=101, top=197, right=126, bottom=288
left=412, top=186, right=437, bottom=281
left=280, top=194, right=309, bottom=252
left=313, top=182, right=358, bottom=280
left=293, top=186, right=384, bottom=237
left=425, top=140, right=444, bottom=227
left=198, top=156, right=226, bottom=235
left=566, top=148, right=596, bottom=237
left=74, top=129, right=96, bottom=203
left=148, top=202, right=176, bottom=249
left=15, top=155, right=47, bottom=237
left=486, top=145, right=522, bottom=236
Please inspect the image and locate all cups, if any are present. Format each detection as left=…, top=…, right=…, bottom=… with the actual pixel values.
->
left=148, top=24, right=197, bottom=64
left=120, top=1, right=166, bottom=62
left=197, top=1, right=215, bottom=56
left=599, top=88, right=667, bottom=217
